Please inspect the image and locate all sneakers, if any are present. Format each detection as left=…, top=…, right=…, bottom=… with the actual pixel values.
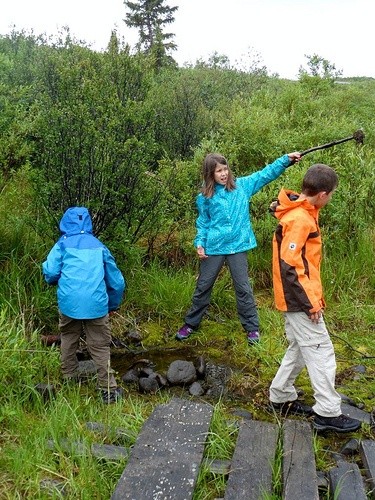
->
left=175, top=323, right=196, bottom=340
left=312, top=410, right=362, bottom=433
left=270, top=400, right=314, bottom=419
left=246, top=331, right=260, bottom=345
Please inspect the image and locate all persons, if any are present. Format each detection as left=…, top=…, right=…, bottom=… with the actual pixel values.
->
left=268, top=163, right=364, bottom=431
left=42, top=206, right=125, bottom=405
left=175, top=150, right=301, bottom=343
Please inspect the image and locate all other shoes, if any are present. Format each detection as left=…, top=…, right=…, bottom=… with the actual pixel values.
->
left=62, top=377, right=84, bottom=389
left=102, top=387, right=124, bottom=404
